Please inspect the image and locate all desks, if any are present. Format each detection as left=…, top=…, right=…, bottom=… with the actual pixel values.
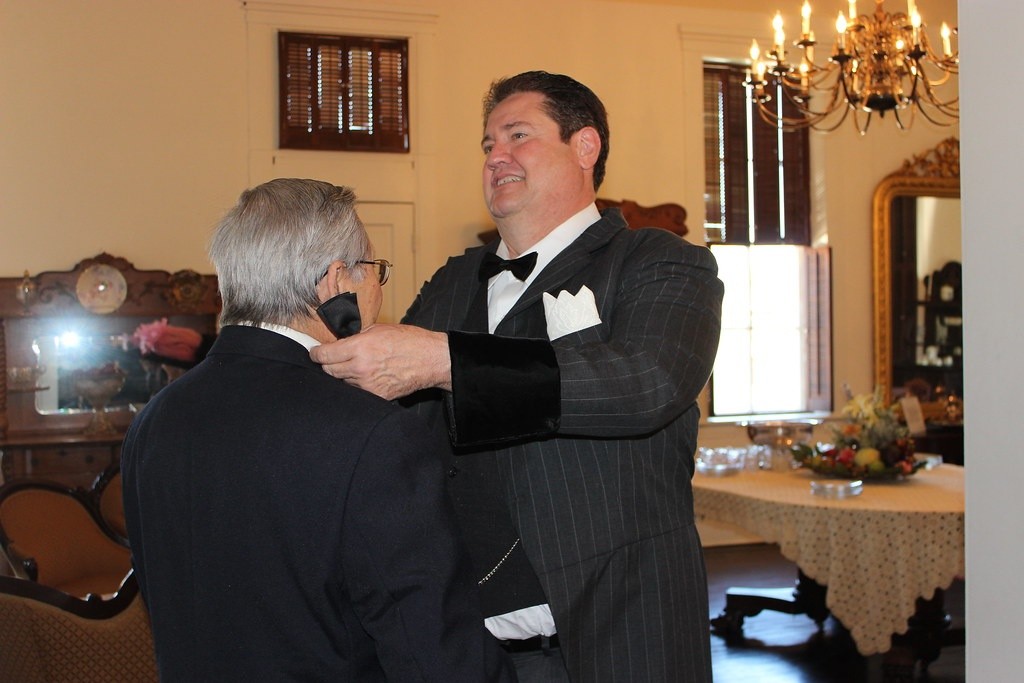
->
left=691, top=457, right=965, bottom=683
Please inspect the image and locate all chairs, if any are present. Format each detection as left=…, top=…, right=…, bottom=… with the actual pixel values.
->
left=1, top=459, right=159, bottom=683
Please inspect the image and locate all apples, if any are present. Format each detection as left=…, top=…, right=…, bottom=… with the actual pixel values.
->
left=812, top=442, right=887, bottom=472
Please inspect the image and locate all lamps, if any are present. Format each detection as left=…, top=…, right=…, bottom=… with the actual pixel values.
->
left=742, top=0, right=959, bottom=136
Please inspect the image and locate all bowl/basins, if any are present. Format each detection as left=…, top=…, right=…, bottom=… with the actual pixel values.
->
left=748, top=421, right=814, bottom=449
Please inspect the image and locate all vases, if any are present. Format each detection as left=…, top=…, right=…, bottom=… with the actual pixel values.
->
left=74, top=377, right=127, bottom=410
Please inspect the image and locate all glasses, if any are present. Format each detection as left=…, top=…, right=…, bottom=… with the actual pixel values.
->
left=323, top=259, right=394, bottom=286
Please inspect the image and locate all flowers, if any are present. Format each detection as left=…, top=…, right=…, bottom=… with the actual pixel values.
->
left=828, top=388, right=909, bottom=448
left=76, top=360, right=128, bottom=377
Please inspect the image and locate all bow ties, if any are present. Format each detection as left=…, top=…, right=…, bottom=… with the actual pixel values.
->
left=477, top=251, right=538, bottom=282
left=315, top=291, right=362, bottom=340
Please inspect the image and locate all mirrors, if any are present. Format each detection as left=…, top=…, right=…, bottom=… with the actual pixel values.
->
left=872, top=136, right=963, bottom=424
left=0, top=252, right=222, bottom=435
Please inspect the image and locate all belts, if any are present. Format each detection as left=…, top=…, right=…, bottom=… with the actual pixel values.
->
left=499, top=634, right=559, bottom=653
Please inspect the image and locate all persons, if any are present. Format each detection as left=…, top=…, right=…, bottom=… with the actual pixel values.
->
left=311, top=71, right=727, bottom=683
left=121, top=178, right=517, bottom=683
